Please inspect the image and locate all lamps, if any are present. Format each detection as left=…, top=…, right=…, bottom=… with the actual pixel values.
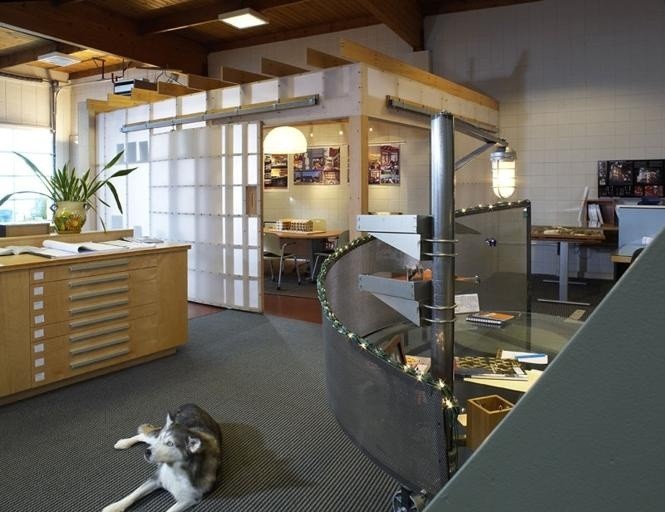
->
left=219, top=7, right=270, bottom=30
left=38, top=51, right=81, bottom=67
left=490, top=152, right=516, bottom=199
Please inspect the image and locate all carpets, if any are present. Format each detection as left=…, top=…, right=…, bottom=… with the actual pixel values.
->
left=264, top=259, right=319, bottom=299
left=0, top=309, right=432, bottom=512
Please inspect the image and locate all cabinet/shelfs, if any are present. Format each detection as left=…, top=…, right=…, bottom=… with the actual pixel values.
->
left=356, top=214, right=459, bottom=327
left=0, top=244, right=192, bottom=406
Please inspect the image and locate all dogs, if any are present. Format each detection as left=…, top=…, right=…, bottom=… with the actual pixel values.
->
left=100, top=403, right=223, bottom=512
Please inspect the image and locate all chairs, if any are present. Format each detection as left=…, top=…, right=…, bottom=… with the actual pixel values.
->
left=263, top=218, right=349, bottom=290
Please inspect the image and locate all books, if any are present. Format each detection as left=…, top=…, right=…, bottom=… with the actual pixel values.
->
left=452, top=348, right=550, bottom=381
left=588, top=203, right=607, bottom=224
left=466, top=310, right=517, bottom=327
left=0, top=235, right=169, bottom=260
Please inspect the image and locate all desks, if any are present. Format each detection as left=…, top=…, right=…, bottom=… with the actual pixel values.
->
left=360, top=310, right=586, bottom=470
left=530, top=225, right=648, bottom=306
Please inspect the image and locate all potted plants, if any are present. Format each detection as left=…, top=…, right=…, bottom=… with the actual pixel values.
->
left=0, top=150, right=137, bottom=236
